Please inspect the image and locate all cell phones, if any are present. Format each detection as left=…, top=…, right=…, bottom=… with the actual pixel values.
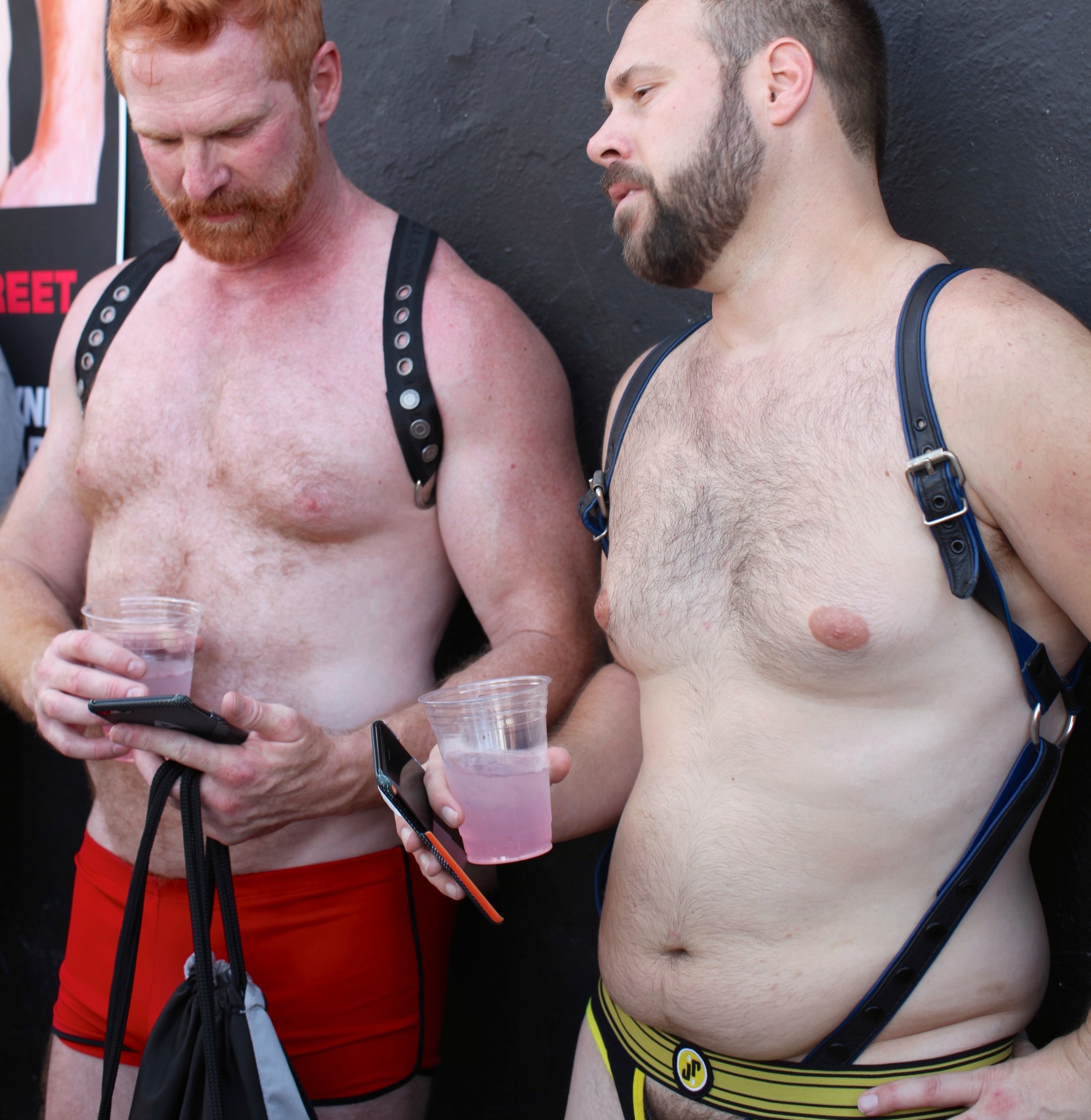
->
left=88, top=694, right=250, bottom=746
left=369, top=720, right=503, bottom=925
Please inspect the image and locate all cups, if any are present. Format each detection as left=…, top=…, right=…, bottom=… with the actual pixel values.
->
left=417, top=676, right=553, bottom=866
left=80, top=597, right=207, bottom=764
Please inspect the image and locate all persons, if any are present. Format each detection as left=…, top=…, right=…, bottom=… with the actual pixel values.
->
left=1, top=0, right=601, bottom=1118
left=0, top=0, right=104, bottom=206
left=388, top=0, right=1091, bottom=1120
